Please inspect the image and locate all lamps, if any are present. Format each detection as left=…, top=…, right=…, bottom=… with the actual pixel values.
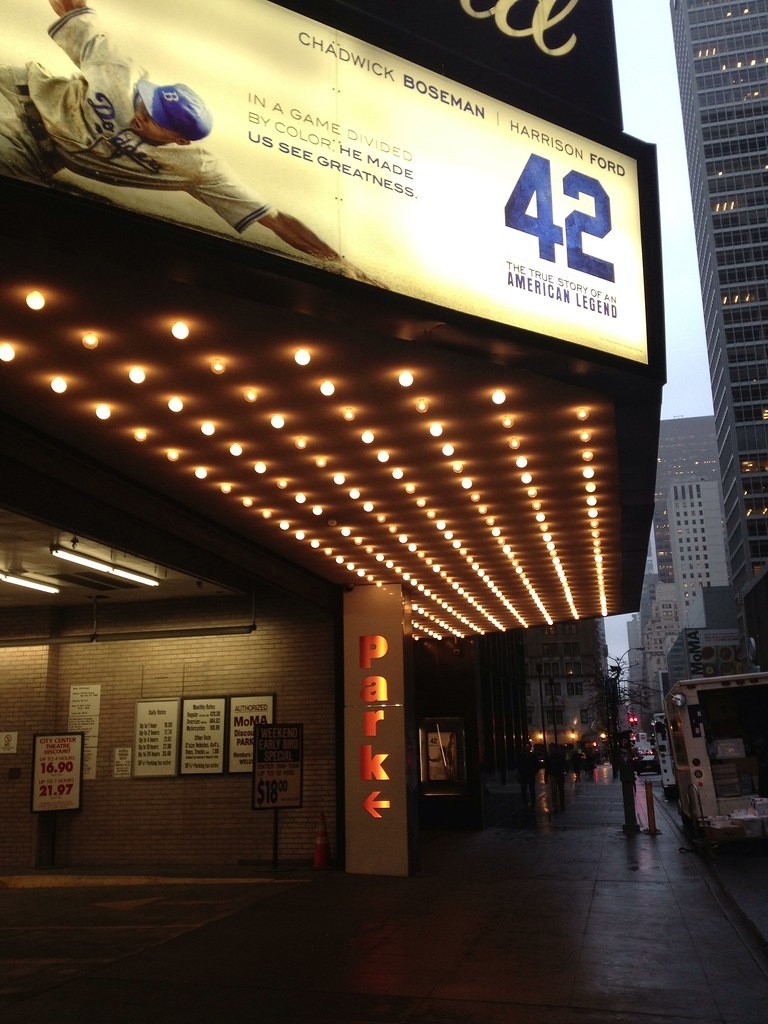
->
left=0, top=569, right=60, bottom=593
left=51, top=547, right=159, bottom=587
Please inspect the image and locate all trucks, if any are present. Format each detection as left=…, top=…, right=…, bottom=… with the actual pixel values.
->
left=630, top=732, right=655, bottom=768
left=650, top=713, right=676, bottom=800
left=661, top=670, right=768, bottom=853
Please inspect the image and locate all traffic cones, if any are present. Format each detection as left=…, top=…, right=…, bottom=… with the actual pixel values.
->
left=307, top=809, right=334, bottom=871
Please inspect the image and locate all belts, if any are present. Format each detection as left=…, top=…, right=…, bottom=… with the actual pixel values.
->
left=16, top=85, right=59, bottom=161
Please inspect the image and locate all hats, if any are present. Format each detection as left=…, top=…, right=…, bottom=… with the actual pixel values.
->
left=137, top=79, right=211, bottom=140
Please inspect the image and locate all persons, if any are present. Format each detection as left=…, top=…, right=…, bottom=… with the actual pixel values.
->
left=519, top=742, right=540, bottom=808
left=564, top=741, right=597, bottom=781
left=0, top=0, right=395, bottom=292
left=650, top=749, right=658, bottom=767
left=544, top=743, right=570, bottom=813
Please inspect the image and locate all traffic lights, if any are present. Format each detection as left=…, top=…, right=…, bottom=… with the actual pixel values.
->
left=634, top=716, right=637, bottom=726
left=630, top=716, right=634, bottom=727
